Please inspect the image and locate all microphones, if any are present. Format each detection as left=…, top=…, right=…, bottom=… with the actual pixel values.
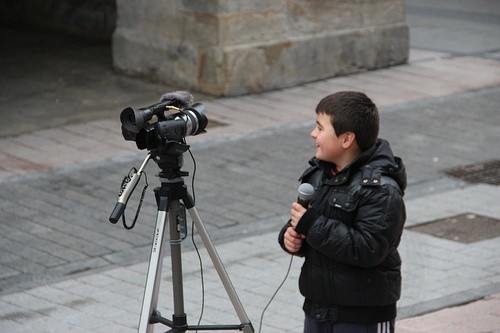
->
left=295, top=183, right=314, bottom=210
left=139, top=90, right=194, bottom=115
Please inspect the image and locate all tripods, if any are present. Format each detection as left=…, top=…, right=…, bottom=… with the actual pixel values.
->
left=135, top=148, right=256, bottom=333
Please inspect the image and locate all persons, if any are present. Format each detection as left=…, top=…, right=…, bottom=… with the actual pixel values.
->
left=278, top=90, right=410, bottom=333
